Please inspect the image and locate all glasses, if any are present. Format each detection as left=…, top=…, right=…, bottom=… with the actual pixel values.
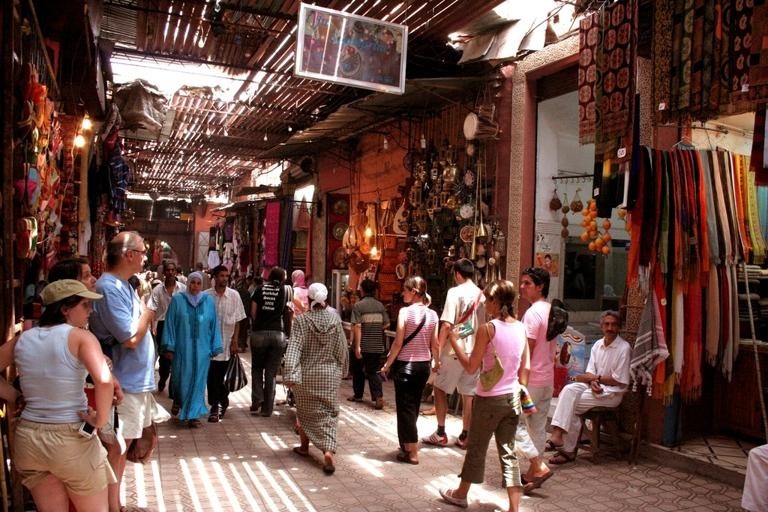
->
left=131, top=250, right=147, bottom=254
left=525, top=267, right=541, bottom=277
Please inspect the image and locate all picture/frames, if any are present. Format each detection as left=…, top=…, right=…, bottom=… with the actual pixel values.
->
left=293, top=2, right=409, bottom=96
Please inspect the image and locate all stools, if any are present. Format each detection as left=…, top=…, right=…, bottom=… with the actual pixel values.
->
left=574, top=407, right=623, bottom=464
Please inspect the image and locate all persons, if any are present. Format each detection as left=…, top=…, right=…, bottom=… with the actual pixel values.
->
left=238, top=275, right=262, bottom=353
left=89, top=231, right=156, bottom=512
left=249, top=266, right=294, bottom=417
left=439, top=279, right=531, bottom=512
left=0, top=278, right=114, bottom=512
left=545, top=310, right=632, bottom=465
left=147, top=261, right=188, bottom=399
left=421, top=258, right=486, bottom=449
left=291, top=270, right=310, bottom=316
left=161, top=272, right=224, bottom=428
left=513, top=267, right=557, bottom=496
left=380, top=275, right=441, bottom=465
left=203, top=265, right=248, bottom=422
left=283, top=282, right=350, bottom=477
left=142, top=260, right=215, bottom=303
left=347, top=279, right=391, bottom=409
left=47, top=258, right=121, bottom=512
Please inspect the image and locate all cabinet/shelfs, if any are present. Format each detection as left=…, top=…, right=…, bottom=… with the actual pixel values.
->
left=712, top=249, right=768, bottom=446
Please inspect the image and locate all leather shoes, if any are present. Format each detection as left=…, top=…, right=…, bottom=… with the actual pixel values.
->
left=158, top=379, right=166, bottom=392
left=169, top=393, right=174, bottom=399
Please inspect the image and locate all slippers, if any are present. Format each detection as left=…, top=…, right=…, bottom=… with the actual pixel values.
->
left=397, top=453, right=418, bottom=464
left=522, top=469, right=553, bottom=493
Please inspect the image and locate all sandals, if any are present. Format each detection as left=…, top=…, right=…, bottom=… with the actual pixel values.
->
left=544, top=440, right=564, bottom=451
left=549, top=447, right=578, bottom=464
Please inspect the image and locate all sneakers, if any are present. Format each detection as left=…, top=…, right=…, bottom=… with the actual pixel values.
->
left=375, top=397, right=384, bottom=409
left=218, top=407, right=226, bottom=419
left=455, top=438, right=467, bottom=449
left=208, top=413, right=218, bottom=422
left=440, top=488, right=468, bottom=508
left=250, top=400, right=264, bottom=413
left=294, top=447, right=308, bottom=456
left=422, top=433, right=447, bottom=445
left=172, top=404, right=179, bottom=415
left=261, top=408, right=272, bottom=416
left=347, top=396, right=362, bottom=401
left=494, top=508, right=504, bottom=512
left=189, top=419, right=201, bottom=427
left=324, top=452, right=335, bottom=473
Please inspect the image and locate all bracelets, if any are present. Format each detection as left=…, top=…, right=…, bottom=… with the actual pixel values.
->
left=15, top=395, right=23, bottom=405
left=595, top=376, right=601, bottom=386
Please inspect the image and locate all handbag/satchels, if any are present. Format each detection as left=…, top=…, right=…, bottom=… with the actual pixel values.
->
left=480, top=356, right=503, bottom=392
left=127, top=422, right=157, bottom=465
left=223, top=352, right=247, bottom=391
left=86, top=338, right=112, bottom=385
left=285, top=285, right=295, bottom=315
left=376, top=349, right=393, bottom=381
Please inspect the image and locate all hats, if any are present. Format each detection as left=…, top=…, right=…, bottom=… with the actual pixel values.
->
left=547, top=299, right=568, bottom=341
left=39, top=279, right=104, bottom=305
left=309, top=283, right=328, bottom=310
